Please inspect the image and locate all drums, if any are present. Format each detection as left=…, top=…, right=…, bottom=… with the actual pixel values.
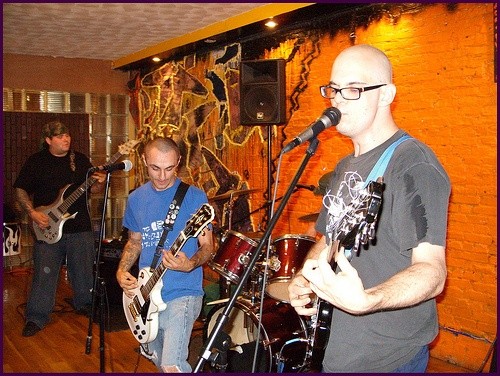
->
left=202, top=296, right=310, bottom=374
left=207, top=228, right=259, bottom=287
left=262, top=233, right=319, bottom=303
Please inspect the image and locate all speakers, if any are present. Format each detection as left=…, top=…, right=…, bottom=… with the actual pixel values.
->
left=239, top=58, right=286, bottom=125
left=95, top=240, right=140, bottom=304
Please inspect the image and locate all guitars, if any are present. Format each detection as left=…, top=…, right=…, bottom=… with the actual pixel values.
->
left=122, top=202, right=215, bottom=345
left=31, top=137, right=141, bottom=245
left=304, top=175, right=385, bottom=372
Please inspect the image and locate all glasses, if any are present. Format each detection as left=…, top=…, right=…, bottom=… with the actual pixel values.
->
left=320, top=84, right=387, bottom=100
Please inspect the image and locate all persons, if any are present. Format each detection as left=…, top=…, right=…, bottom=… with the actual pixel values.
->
left=117, top=138, right=219, bottom=372
left=16, top=119, right=107, bottom=336
left=287, top=44, right=450, bottom=373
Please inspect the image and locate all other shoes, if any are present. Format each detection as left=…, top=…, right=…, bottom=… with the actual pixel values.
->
left=22, top=321, right=40, bottom=337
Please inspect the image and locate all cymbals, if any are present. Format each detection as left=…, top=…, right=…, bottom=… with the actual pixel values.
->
left=298, top=213, right=320, bottom=222
left=208, top=188, right=264, bottom=200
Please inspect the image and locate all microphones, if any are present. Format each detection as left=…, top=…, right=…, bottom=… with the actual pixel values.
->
left=91, top=160, right=132, bottom=173
left=283, top=107, right=341, bottom=155
left=296, top=184, right=315, bottom=191
